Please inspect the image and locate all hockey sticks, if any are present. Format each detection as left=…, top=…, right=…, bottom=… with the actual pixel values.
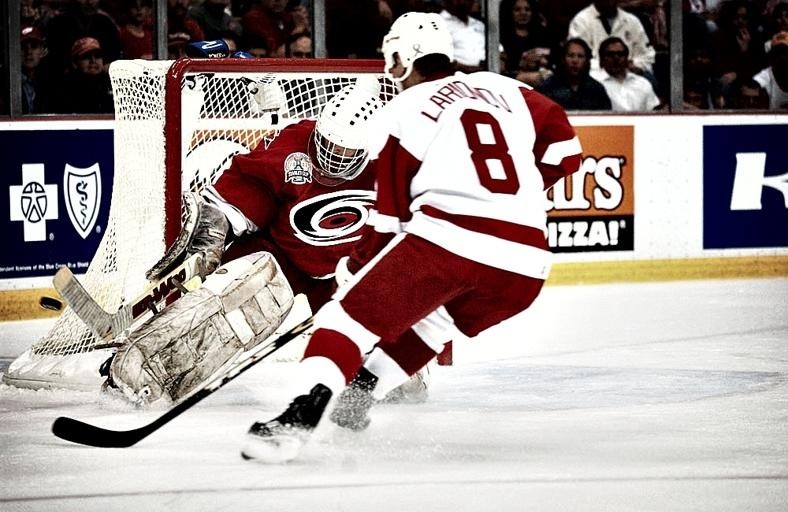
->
left=52, top=252, right=203, bottom=343
left=52, top=315, right=313, bottom=448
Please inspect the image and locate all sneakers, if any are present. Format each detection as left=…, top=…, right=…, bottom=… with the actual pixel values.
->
left=390, top=377, right=427, bottom=403
left=331, top=391, right=372, bottom=430
left=247, top=394, right=330, bottom=445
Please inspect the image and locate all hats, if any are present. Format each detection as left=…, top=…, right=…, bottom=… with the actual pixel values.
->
left=21, top=26, right=42, bottom=46
left=70, top=38, right=99, bottom=61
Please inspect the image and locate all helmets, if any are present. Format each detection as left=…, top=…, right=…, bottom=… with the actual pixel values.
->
left=315, top=86, right=389, bottom=180
left=382, top=11, right=455, bottom=82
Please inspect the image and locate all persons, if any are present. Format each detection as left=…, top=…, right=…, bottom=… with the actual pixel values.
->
left=98, top=83, right=434, bottom=407
left=236, top=8, right=582, bottom=469
left=1, top=0, right=788, bottom=118
